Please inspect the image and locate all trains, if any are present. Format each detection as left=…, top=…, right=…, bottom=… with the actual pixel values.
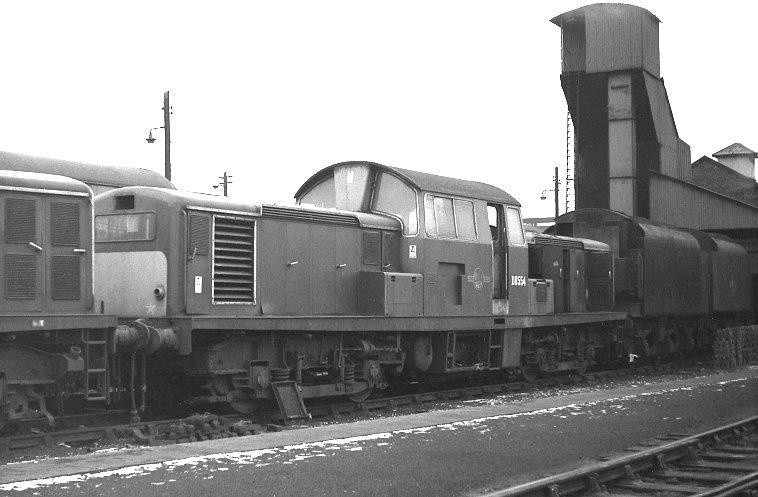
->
left=0, top=149, right=756, bottom=431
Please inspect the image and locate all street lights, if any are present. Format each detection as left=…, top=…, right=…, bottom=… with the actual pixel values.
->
left=212, top=172, right=228, bottom=196
left=147, top=90, right=172, bottom=182
left=540, top=165, right=560, bottom=217
